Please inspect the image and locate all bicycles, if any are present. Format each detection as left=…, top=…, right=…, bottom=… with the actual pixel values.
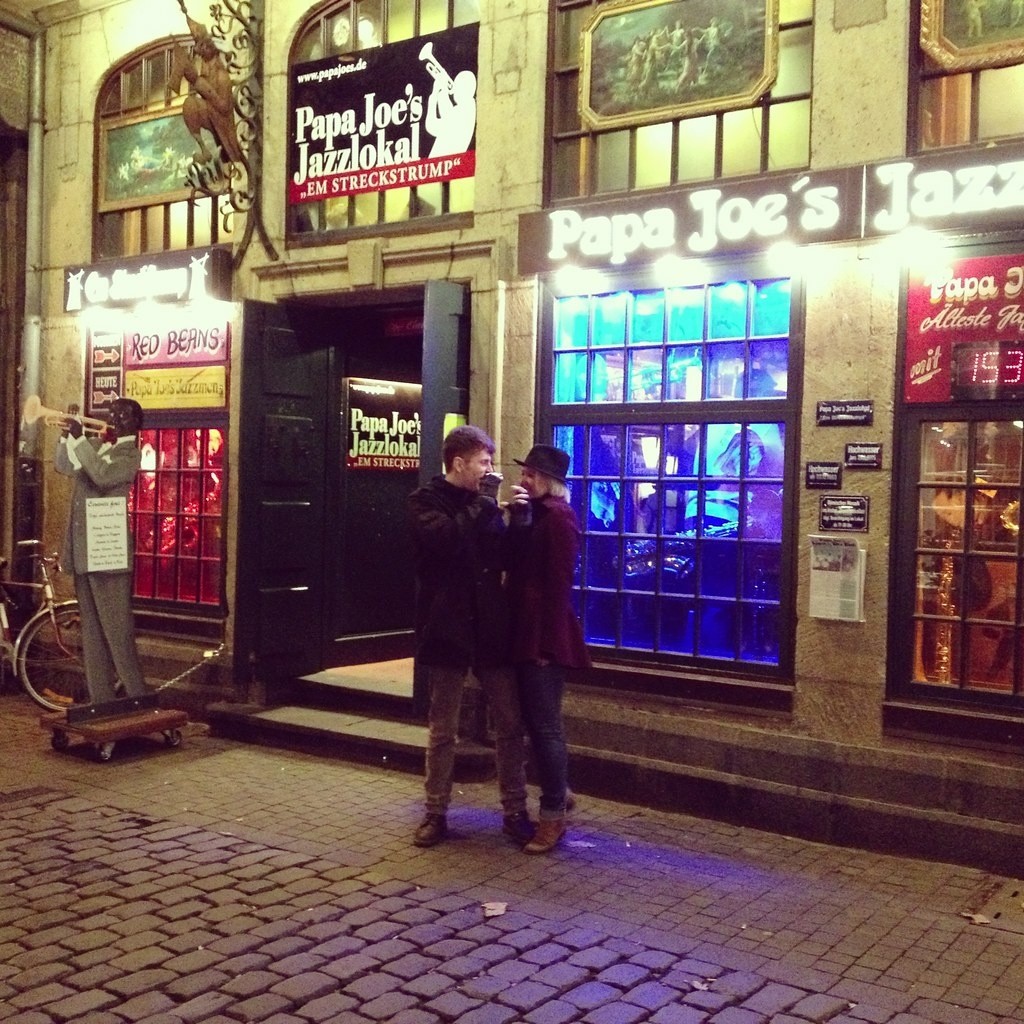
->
left=0, top=540, right=125, bottom=713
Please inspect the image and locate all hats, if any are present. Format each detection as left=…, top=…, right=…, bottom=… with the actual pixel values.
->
left=512, top=444, right=571, bottom=483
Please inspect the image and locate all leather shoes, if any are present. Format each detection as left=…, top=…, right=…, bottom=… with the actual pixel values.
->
left=523, top=809, right=567, bottom=856
left=413, top=812, right=448, bottom=847
left=502, top=810, right=537, bottom=846
left=565, top=788, right=578, bottom=813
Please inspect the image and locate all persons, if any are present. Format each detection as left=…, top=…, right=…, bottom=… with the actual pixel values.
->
left=55, top=399, right=150, bottom=704
left=402, top=425, right=595, bottom=857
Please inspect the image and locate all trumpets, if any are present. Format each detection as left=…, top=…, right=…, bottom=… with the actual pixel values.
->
left=22, top=394, right=117, bottom=435
